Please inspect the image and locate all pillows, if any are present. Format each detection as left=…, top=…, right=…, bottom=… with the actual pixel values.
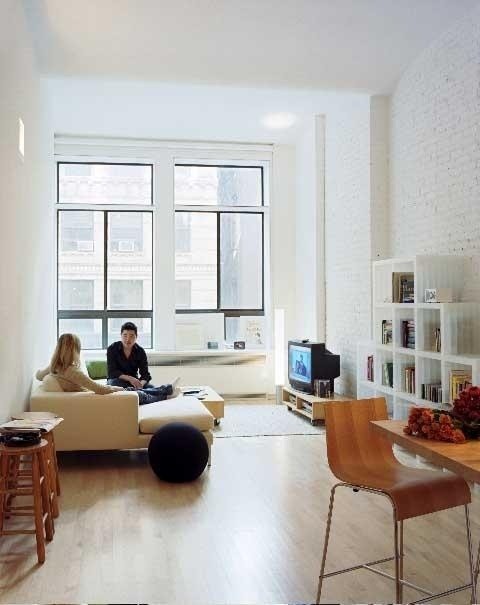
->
left=42, top=374, right=64, bottom=391
left=85, top=360, right=108, bottom=379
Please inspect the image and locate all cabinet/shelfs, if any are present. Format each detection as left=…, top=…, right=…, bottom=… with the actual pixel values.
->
left=356, top=255, right=480, bottom=421
left=281, top=386, right=355, bottom=426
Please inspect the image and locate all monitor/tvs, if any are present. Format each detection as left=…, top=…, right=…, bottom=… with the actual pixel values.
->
left=288, top=339, right=340, bottom=395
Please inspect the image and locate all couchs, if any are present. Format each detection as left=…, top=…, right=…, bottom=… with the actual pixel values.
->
left=30, top=358, right=224, bottom=466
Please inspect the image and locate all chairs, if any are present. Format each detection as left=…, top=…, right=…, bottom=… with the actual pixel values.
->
left=316, top=396, right=480, bottom=605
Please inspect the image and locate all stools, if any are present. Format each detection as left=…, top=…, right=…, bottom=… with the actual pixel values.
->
left=0, top=438, right=54, bottom=564
left=0, top=411, right=60, bottom=533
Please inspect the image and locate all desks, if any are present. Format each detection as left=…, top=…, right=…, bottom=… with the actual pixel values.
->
left=369, top=420, right=480, bottom=485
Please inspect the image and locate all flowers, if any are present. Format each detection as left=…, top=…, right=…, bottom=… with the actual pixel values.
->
left=403, top=386, right=480, bottom=443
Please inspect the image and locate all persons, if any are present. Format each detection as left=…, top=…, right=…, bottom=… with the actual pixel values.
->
left=107, top=322, right=152, bottom=388
left=36, top=334, right=181, bottom=405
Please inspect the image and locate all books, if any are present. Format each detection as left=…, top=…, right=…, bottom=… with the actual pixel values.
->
left=430, top=326, right=441, bottom=352
left=0, top=417, right=65, bottom=434
left=381, top=320, right=393, bottom=344
left=449, top=369, right=472, bottom=402
left=401, top=319, right=415, bottom=348
left=392, top=272, right=414, bottom=303
left=382, top=363, right=393, bottom=387
left=421, top=384, right=442, bottom=402
left=367, top=355, right=374, bottom=381
left=405, top=368, right=415, bottom=394
